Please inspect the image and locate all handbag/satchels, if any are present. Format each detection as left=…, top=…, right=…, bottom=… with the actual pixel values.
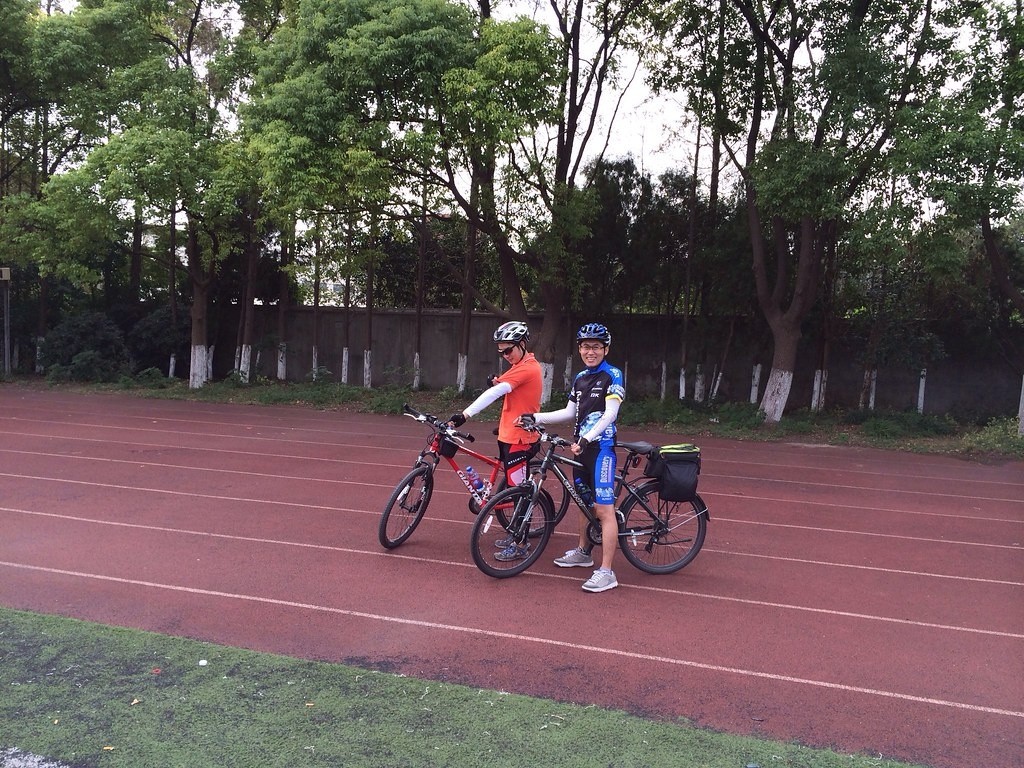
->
left=658, top=460, right=698, bottom=502
left=643, top=442, right=701, bottom=477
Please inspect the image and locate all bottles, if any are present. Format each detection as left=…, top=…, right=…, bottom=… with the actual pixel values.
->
left=466, top=465, right=485, bottom=491
left=575, top=477, right=595, bottom=507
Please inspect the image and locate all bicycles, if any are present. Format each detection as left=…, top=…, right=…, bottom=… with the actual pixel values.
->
left=377, top=401, right=570, bottom=549
left=468, top=415, right=710, bottom=580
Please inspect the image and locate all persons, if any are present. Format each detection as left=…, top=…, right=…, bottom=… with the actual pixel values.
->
left=513, top=323, right=625, bottom=593
left=444, top=321, right=543, bottom=562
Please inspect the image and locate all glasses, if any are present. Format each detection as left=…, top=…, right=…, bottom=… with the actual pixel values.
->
left=498, top=344, right=518, bottom=355
left=580, top=344, right=606, bottom=350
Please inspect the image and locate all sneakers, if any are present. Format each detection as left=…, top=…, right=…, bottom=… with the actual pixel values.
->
left=554, top=547, right=594, bottom=567
left=493, top=534, right=531, bottom=560
left=581, top=569, right=618, bottom=592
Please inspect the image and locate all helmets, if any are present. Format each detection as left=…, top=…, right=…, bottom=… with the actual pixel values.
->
left=576, top=323, right=611, bottom=347
left=493, top=320, right=531, bottom=344
